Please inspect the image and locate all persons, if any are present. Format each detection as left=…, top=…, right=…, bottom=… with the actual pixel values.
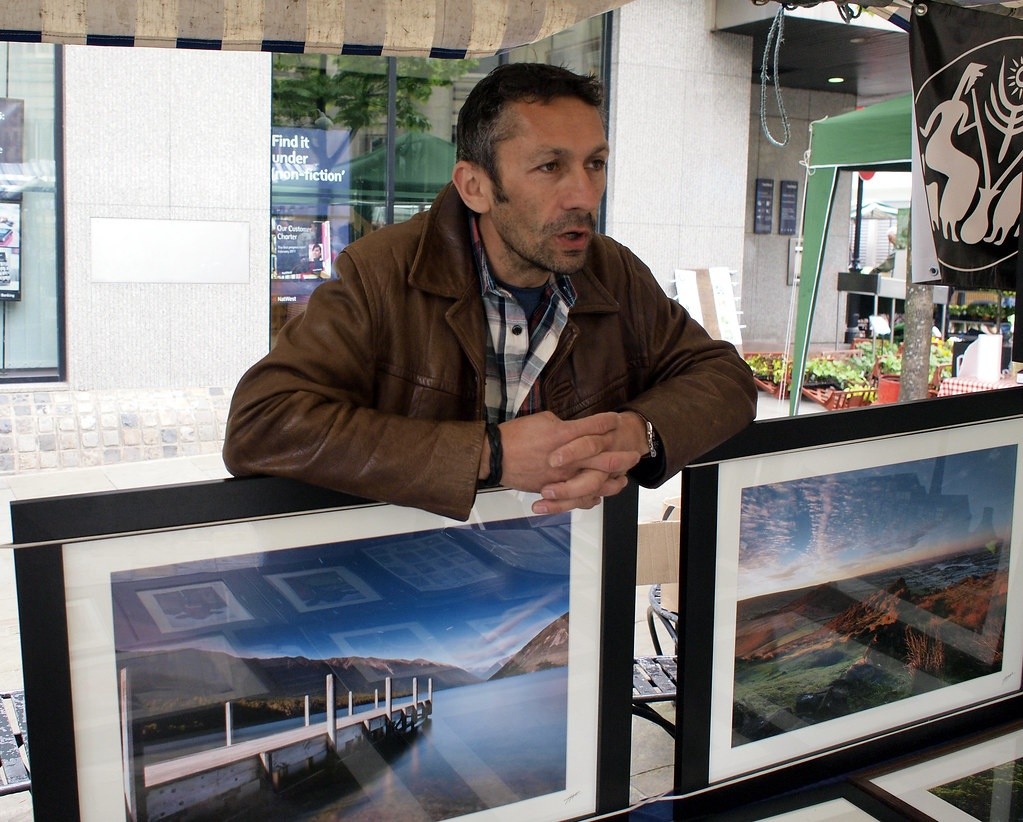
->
left=311, top=245, right=322, bottom=260
left=221, top=62, right=759, bottom=522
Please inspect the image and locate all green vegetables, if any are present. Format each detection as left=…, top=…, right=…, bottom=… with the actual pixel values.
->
left=744, top=336, right=956, bottom=402
left=946, top=302, right=1017, bottom=319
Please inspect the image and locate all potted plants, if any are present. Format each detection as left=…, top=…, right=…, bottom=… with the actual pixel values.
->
left=744, top=301, right=1016, bottom=392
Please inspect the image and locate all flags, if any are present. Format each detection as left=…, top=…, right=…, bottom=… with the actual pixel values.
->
left=908, top=2, right=1023, bottom=294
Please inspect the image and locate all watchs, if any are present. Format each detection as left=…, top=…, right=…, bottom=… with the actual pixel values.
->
left=633, top=410, right=658, bottom=457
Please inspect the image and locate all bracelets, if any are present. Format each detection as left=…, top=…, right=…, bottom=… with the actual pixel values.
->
left=479, top=422, right=502, bottom=490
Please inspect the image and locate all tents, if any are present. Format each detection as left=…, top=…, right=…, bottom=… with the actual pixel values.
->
left=782, top=96, right=912, bottom=419
